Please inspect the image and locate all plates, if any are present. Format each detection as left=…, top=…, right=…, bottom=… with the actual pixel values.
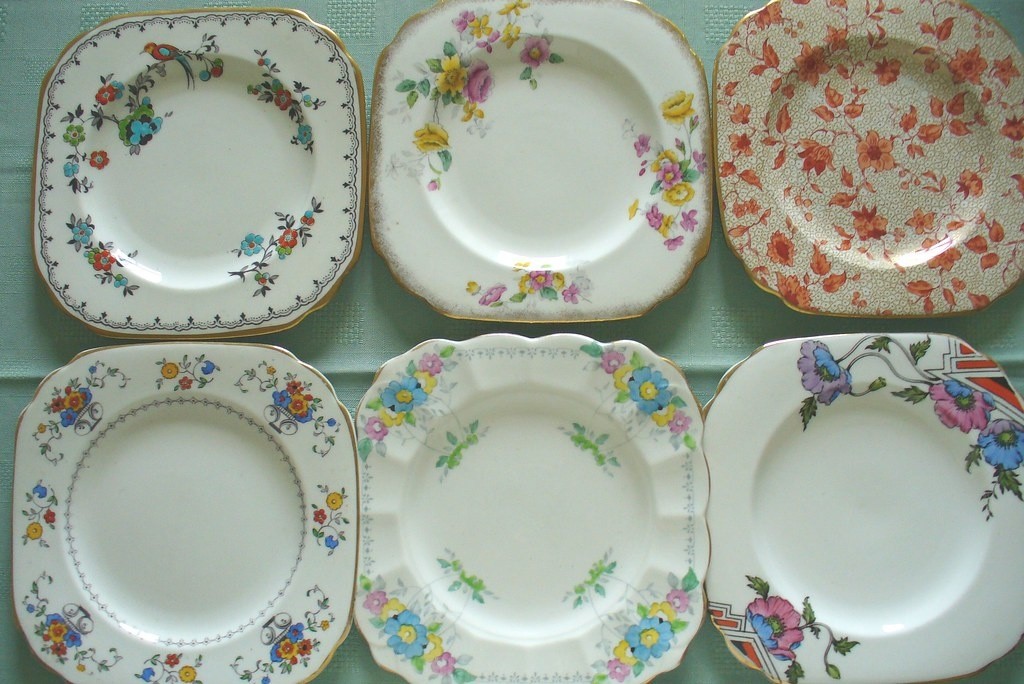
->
left=11, top=341, right=361, bottom=684
left=701, top=332, right=1024, bottom=684
left=710, top=2, right=1024, bottom=320
left=369, top=0, right=711, bottom=323
left=30, top=6, right=367, bottom=341
left=356, top=330, right=712, bottom=684
